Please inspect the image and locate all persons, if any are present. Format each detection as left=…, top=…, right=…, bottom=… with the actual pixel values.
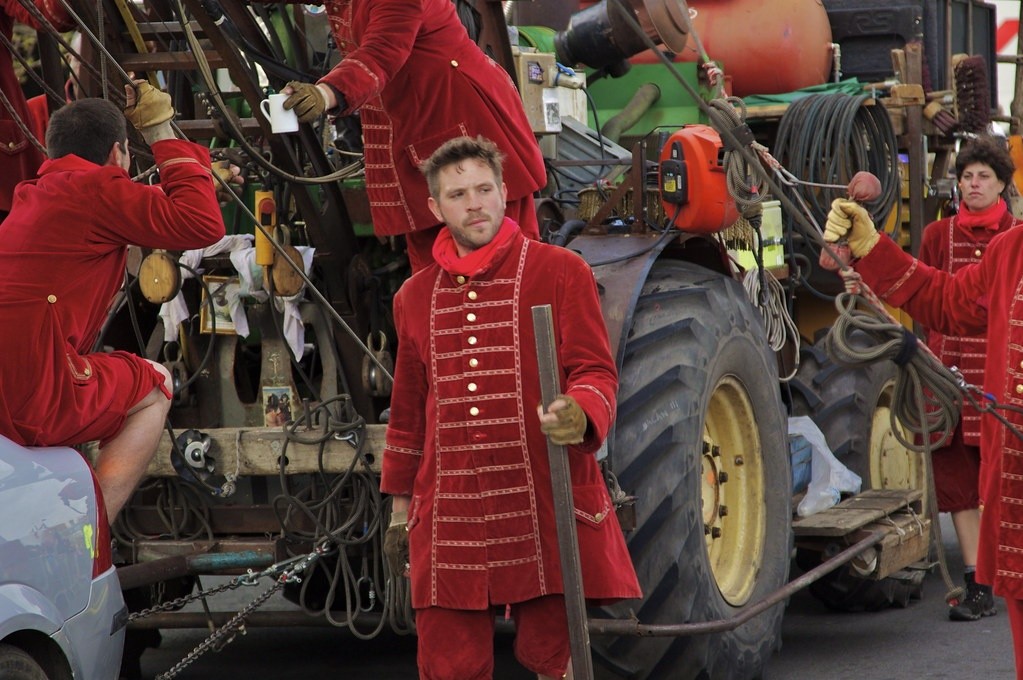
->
left=284, top=0, right=547, bottom=273
left=914, top=141, right=1023, bottom=621
left=0, top=0, right=226, bottom=525
left=380, top=137, right=642, bottom=680
left=822, top=199, right=1023, bottom=680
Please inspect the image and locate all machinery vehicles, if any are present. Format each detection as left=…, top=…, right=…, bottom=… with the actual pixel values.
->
left=0, top=0, right=1023, bottom=680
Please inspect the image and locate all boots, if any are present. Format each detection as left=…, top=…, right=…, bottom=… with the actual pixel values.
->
left=949, top=572, right=996, bottom=620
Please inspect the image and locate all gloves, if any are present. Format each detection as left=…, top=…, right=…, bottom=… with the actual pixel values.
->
left=823, top=197, right=881, bottom=260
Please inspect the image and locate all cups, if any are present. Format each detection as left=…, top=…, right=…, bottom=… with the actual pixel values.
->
left=260, top=93, right=299, bottom=134
